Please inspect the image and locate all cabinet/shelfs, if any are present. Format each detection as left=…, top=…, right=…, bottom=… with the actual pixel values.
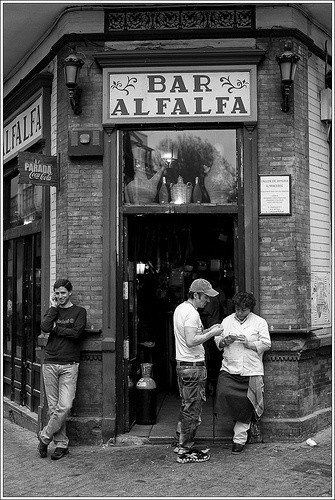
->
left=136, top=264, right=235, bottom=392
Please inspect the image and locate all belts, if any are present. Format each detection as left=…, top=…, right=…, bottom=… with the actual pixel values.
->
left=175, top=361, right=205, bottom=366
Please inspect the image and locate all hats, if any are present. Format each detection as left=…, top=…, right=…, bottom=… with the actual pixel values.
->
left=189, top=278, right=219, bottom=297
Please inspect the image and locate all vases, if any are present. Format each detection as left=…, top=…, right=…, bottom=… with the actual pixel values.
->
left=136, top=363, right=158, bottom=425
left=203, top=144, right=235, bottom=204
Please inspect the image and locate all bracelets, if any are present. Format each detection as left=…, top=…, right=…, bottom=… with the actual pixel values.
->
left=222, top=340, right=226, bottom=346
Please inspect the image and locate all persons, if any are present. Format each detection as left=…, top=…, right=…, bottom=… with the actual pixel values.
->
left=214, top=291, right=272, bottom=452
left=172, top=278, right=224, bottom=463
left=38, top=278, right=86, bottom=459
left=197, top=278, right=227, bottom=397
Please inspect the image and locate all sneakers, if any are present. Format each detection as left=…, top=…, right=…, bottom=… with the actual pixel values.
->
left=174, top=443, right=210, bottom=454
left=37, top=431, right=49, bottom=458
left=51, top=447, right=70, bottom=460
left=176, top=448, right=210, bottom=463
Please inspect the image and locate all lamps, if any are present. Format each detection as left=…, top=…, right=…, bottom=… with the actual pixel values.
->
left=59, top=45, right=86, bottom=115
left=275, top=42, right=301, bottom=113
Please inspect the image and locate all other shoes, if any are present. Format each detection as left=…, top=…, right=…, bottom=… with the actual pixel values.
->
left=232, top=443, right=244, bottom=454
